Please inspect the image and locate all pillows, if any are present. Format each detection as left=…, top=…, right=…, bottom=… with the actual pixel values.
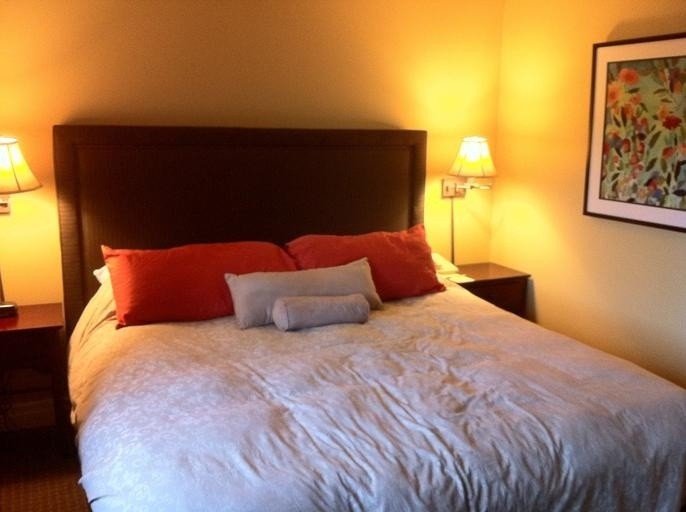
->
left=224, top=257, right=386, bottom=330
left=100, top=241, right=297, bottom=330
left=272, top=292, right=370, bottom=330
left=286, top=224, right=446, bottom=299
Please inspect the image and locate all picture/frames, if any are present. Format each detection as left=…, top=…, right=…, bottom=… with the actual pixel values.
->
left=581, top=31, right=686, bottom=232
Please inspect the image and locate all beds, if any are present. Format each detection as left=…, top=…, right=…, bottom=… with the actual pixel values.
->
left=53, top=127, right=686, bottom=512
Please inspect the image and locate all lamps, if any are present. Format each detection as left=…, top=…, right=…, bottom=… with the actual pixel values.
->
left=0, top=137, right=42, bottom=214
left=440, top=132, right=497, bottom=198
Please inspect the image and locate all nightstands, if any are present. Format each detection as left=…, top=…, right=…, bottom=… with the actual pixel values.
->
left=440, top=261, right=533, bottom=320
left=0, top=303, right=68, bottom=462
